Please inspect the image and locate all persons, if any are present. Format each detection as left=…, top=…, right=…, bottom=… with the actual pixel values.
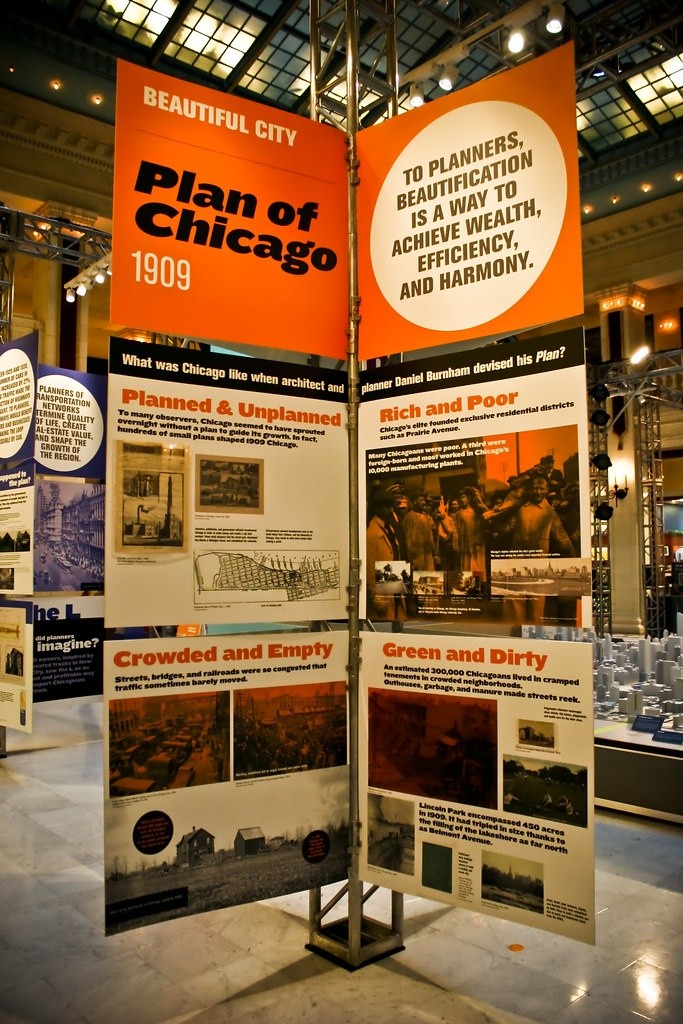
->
left=367, top=483, right=504, bottom=620
left=503, top=790, right=519, bottom=804
left=557, top=793, right=573, bottom=816
left=480, top=454, right=579, bottom=626
left=544, top=792, right=552, bottom=808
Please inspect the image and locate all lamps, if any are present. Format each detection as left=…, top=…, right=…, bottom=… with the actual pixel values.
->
left=64, top=252, right=111, bottom=303
left=545, top=0, right=565, bottom=33
left=407, top=61, right=439, bottom=107
left=501, top=2, right=542, bottom=54
left=436, top=43, right=470, bottom=89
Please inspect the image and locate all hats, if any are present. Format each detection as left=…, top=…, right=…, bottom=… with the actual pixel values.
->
left=545, top=456, right=555, bottom=463
left=386, top=484, right=400, bottom=495
